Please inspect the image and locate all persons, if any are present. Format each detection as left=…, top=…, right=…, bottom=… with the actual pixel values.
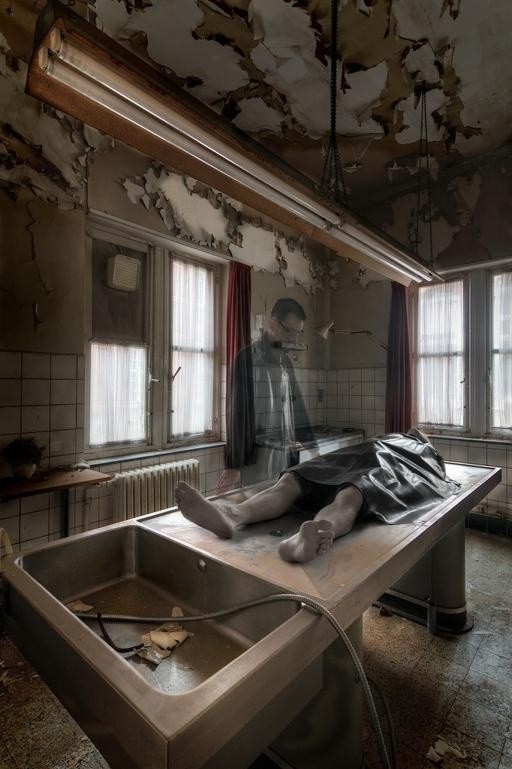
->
left=175, top=424, right=441, bottom=567
left=218, top=295, right=325, bottom=494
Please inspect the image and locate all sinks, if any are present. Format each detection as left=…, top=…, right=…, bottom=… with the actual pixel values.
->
left=13, top=524, right=304, bottom=696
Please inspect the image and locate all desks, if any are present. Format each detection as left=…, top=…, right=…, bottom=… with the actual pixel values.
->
left=0, top=465, right=111, bottom=547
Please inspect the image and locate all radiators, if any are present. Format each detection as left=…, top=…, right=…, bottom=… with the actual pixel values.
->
left=108, top=457, right=200, bottom=525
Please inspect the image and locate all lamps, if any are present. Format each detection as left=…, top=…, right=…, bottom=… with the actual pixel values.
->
left=313, top=319, right=388, bottom=353
left=26, top=0, right=338, bottom=237
left=313, top=45, right=445, bottom=289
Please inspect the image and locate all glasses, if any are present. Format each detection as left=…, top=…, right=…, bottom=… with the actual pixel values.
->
left=279, top=321, right=304, bottom=337
